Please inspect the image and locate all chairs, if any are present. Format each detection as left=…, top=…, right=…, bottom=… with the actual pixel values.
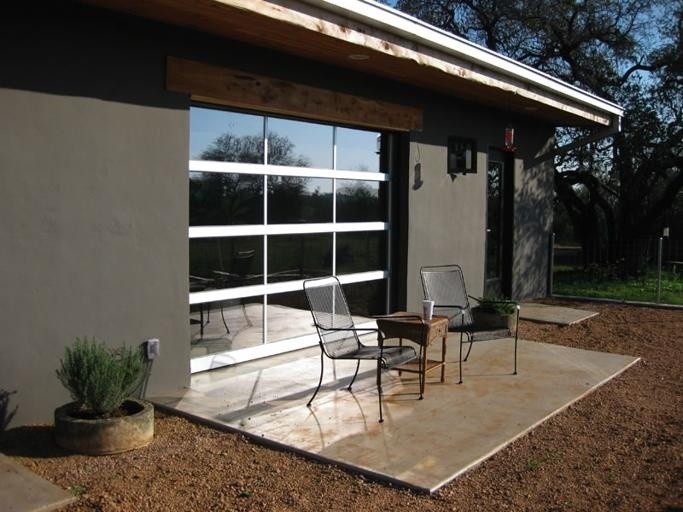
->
left=203, top=247, right=257, bottom=335
left=298, top=273, right=424, bottom=424
left=416, top=263, right=521, bottom=386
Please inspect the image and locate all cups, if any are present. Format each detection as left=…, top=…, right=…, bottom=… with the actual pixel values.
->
left=422, top=299, right=435, bottom=321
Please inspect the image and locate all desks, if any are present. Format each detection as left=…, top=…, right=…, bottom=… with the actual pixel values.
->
left=373, top=309, right=449, bottom=398
left=188, top=272, right=221, bottom=337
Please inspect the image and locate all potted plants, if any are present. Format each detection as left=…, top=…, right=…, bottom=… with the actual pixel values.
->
left=50, top=337, right=162, bottom=456
